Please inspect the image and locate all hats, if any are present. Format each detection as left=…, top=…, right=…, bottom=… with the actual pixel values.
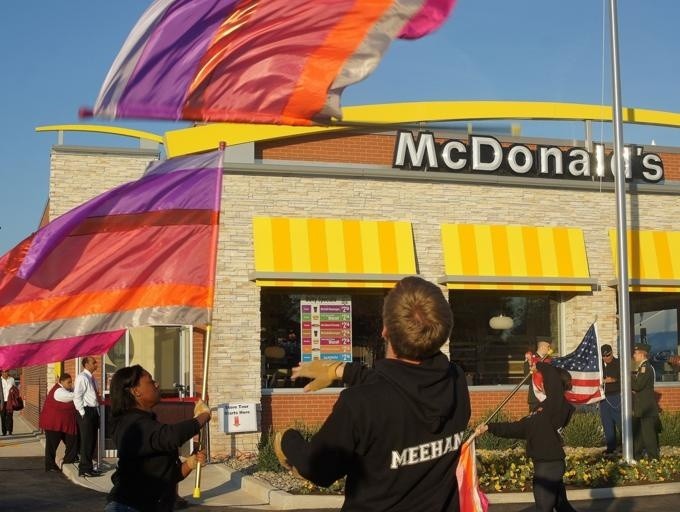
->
left=633, top=342, right=652, bottom=354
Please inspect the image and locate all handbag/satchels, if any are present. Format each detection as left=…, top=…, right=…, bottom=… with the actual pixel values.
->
left=11, top=389, right=24, bottom=411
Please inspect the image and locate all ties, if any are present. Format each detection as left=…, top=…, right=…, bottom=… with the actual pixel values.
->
left=93, top=381, right=103, bottom=405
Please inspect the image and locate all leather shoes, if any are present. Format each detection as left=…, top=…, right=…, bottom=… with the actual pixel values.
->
left=78, top=469, right=99, bottom=477
left=45, top=466, right=61, bottom=474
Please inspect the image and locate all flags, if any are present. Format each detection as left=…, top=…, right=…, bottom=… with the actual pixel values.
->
left=0, top=140, right=228, bottom=373
left=549, top=323, right=606, bottom=406
left=82, top=1, right=459, bottom=133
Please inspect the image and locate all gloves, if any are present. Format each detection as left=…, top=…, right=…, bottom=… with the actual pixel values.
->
left=290, top=358, right=343, bottom=393
left=274, top=430, right=292, bottom=467
left=193, top=399, right=212, bottom=423
left=185, top=451, right=207, bottom=469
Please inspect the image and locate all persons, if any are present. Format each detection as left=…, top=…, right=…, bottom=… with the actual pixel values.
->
left=523, top=335, right=555, bottom=414
left=627, top=341, right=662, bottom=462
left=72, top=355, right=105, bottom=478
left=597, top=344, right=623, bottom=453
left=101, top=364, right=212, bottom=512
left=0, top=368, right=18, bottom=436
left=272, top=274, right=473, bottom=510
left=474, top=358, right=577, bottom=511
left=37, top=372, right=81, bottom=472
